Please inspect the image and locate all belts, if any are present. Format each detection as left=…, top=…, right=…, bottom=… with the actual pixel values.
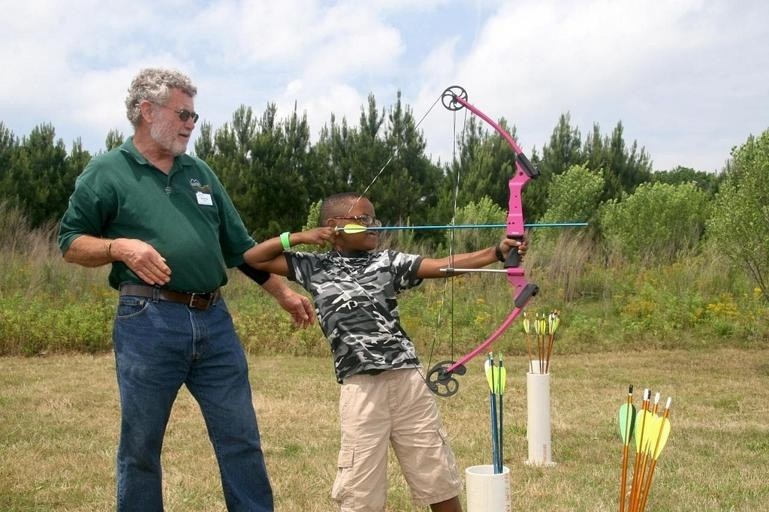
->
left=118, top=283, right=224, bottom=309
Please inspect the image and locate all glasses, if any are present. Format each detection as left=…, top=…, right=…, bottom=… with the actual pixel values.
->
left=330, top=215, right=383, bottom=228
left=147, top=98, right=200, bottom=127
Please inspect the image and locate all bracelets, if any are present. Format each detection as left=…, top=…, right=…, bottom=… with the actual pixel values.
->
left=105, top=240, right=114, bottom=261
left=495, top=244, right=505, bottom=262
left=279, top=231, right=291, bottom=252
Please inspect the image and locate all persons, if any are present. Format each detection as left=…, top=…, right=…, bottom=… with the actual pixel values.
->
left=57, top=70, right=316, bottom=512
left=243, top=192, right=529, bottom=512
